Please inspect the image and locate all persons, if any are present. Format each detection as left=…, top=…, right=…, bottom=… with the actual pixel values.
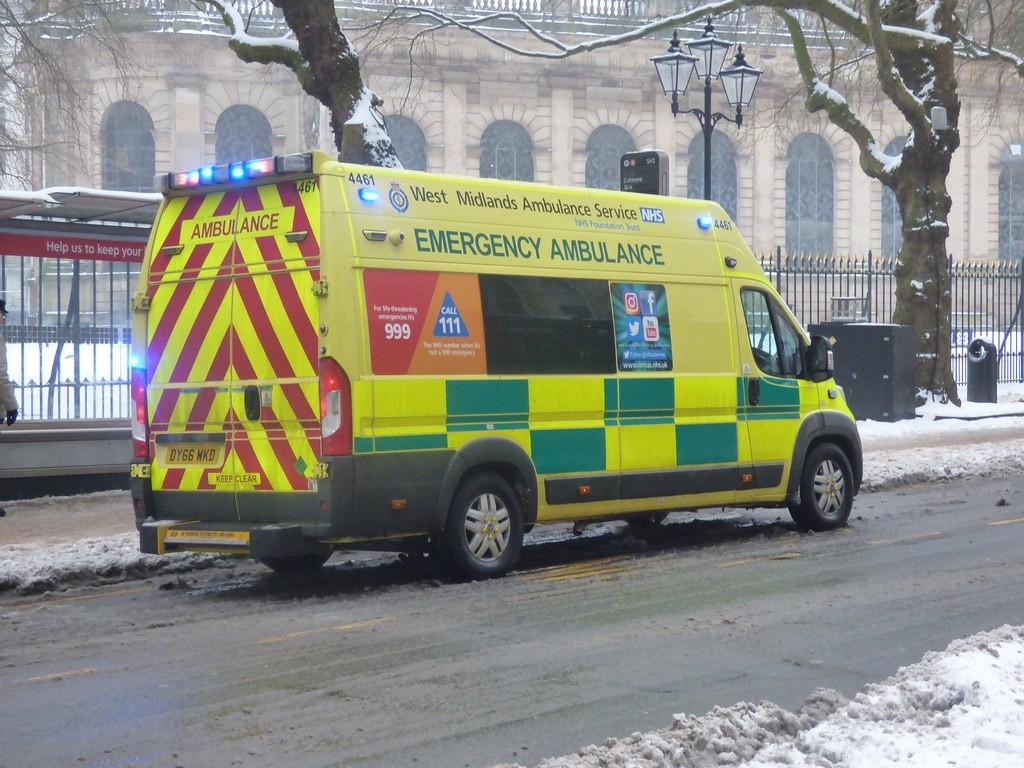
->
left=0, top=300, right=19, bottom=517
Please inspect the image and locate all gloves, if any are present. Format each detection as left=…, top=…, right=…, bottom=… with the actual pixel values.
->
left=6, top=408, right=19, bottom=426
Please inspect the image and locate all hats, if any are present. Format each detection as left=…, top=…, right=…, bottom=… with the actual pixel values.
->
left=0, top=300, right=8, bottom=313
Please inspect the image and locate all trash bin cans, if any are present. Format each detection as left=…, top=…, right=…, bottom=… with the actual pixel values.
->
left=967, top=336, right=997, bottom=403
left=830, top=294, right=872, bottom=325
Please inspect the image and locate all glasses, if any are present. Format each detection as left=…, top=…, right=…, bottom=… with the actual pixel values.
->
left=0, top=311, right=7, bottom=318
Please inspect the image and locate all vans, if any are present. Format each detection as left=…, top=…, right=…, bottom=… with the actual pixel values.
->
left=127, top=149, right=862, bottom=580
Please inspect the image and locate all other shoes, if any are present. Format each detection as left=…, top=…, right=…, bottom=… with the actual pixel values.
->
left=0, top=507, right=6, bottom=516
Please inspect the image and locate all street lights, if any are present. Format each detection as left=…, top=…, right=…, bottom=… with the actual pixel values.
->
left=648, top=15, right=765, bottom=201
